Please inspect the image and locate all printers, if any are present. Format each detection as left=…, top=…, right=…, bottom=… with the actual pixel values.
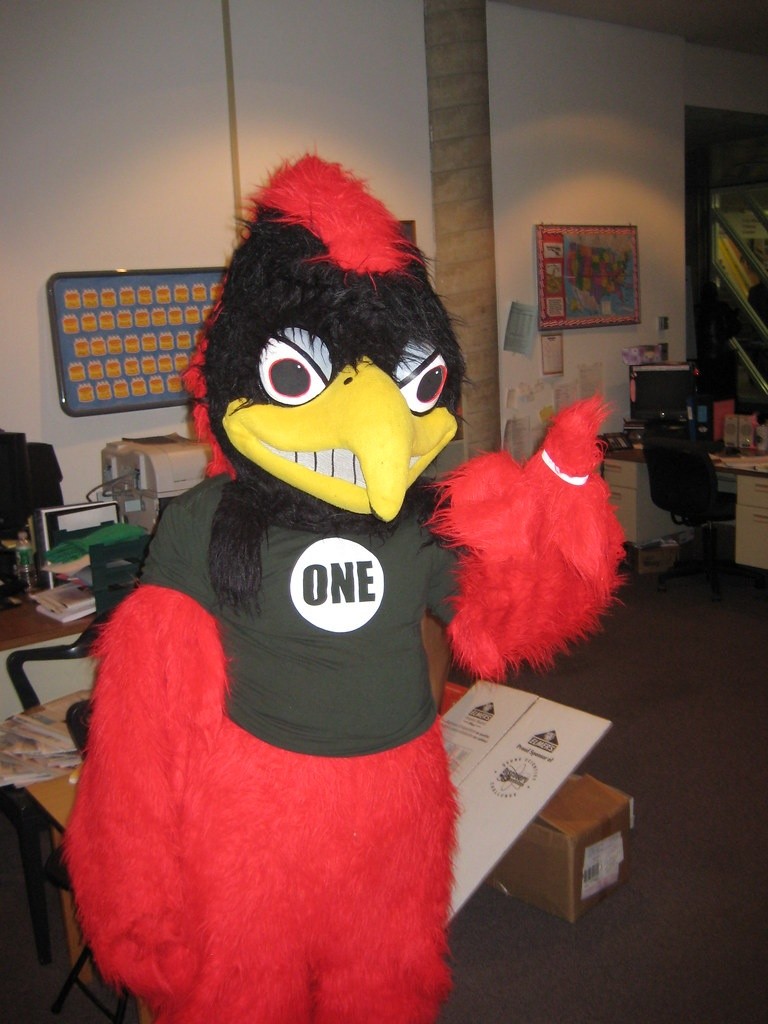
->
left=100, top=431, right=211, bottom=498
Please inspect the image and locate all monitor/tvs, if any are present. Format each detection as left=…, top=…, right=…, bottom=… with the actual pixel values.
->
left=629, top=362, right=696, bottom=423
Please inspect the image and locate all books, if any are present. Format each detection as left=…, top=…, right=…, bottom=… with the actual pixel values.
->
left=31, top=581, right=96, bottom=624
left=1, top=690, right=92, bottom=789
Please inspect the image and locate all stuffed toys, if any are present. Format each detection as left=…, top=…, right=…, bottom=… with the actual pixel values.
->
left=66, top=151, right=623, bottom=1024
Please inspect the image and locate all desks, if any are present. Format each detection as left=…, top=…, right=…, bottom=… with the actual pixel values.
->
left=601, top=449, right=768, bottom=570
left=0, top=594, right=97, bottom=721
left=5, top=686, right=153, bottom=1024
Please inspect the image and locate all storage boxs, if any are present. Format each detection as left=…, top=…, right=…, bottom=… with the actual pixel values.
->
left=623, top=540, right=679, bottom=575
left=483, top=772, right=636, bottom=924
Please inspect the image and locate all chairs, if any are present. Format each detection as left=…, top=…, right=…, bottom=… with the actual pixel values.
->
left=52, top=700, right=130, bottom=1024
left=0, top=608, right=132, bottom=967
left=642, top=442, right=765, bottom=602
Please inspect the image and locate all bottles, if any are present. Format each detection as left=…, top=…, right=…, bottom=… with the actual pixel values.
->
left=15, top=532, right=38, bottom=595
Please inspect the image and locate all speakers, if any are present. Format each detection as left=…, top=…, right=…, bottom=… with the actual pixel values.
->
left=723, top=415, right=739, bottom=449
left=738, top=415, right=755, bottom=448
